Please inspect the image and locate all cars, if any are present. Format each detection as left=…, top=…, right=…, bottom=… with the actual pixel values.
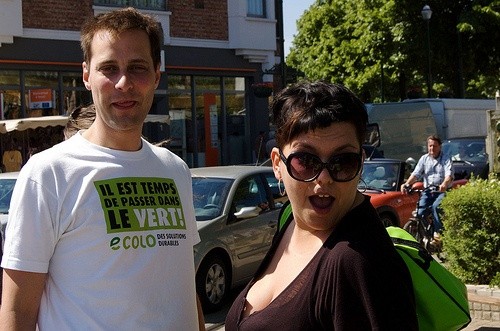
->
left=0, top=172, right=19, bottom=255
left=189, top=165, right=289, bottom=314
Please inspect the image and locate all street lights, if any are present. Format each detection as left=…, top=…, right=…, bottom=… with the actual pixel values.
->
left=421, top=4, right=434, bottom=98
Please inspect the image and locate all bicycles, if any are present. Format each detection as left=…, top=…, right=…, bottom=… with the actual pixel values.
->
left=402, top=185, right=446, bottom=255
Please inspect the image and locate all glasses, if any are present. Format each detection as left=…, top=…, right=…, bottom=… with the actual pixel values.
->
left=280, top=148, right=363, bottom=182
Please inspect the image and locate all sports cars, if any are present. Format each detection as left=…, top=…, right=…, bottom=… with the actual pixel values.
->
left=358, top=158, right=469, bottom=230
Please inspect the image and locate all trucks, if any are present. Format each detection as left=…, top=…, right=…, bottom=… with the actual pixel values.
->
left=360, top=95, right=500, bottom=180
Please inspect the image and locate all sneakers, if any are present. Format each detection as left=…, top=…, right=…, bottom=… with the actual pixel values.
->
left=426, top=238, right=443, bottom=250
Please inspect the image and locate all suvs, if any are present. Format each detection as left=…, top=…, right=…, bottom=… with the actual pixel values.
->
left=438, top=136, right=490, bottom=181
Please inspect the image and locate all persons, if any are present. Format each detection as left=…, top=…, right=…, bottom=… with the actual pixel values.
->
left=225, top=79, right=415, bottom=331
left=62, top=105, right=96, bottom=144
left=0, top=7, right=201, bottom=331
left=401, top=136, right=455, bottom=248
